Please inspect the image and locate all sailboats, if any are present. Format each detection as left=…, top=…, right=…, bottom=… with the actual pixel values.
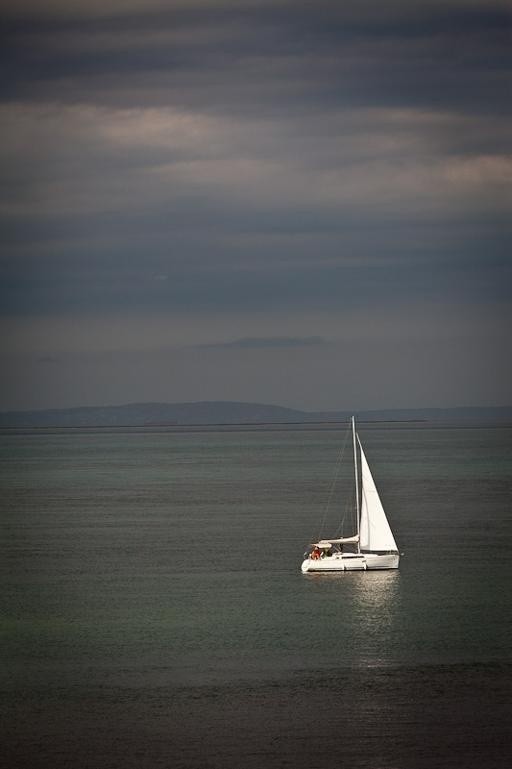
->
left=300, top=412, right=402, bottom=573
